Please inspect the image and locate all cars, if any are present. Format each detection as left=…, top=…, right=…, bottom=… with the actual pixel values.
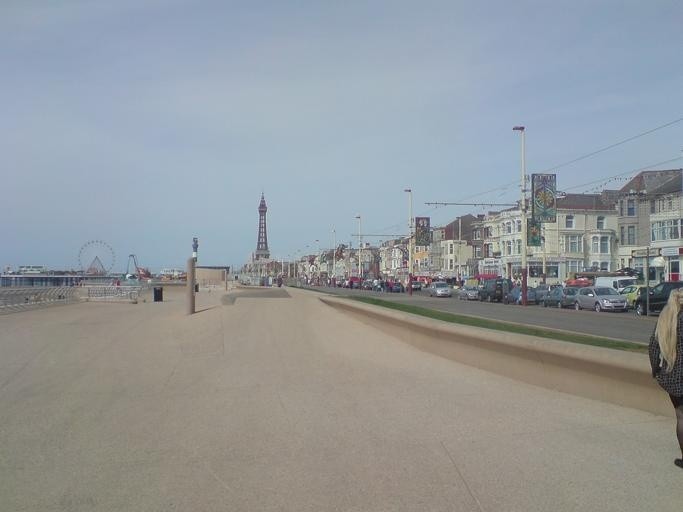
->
left=429, top=282, right=452, bottom=297
left=308, top=276, right=401, bottom=293
left=619, top=285, right=653, bottom=310
left=456, top=285, right=480, bottom=301
left=503, top=285, right=538, bottom=307
left=533, top=284, right=563, bottom=305
left=573, top=286, right=627, bottom=313
left=405, top=281, right=421, bottom=291
left=543, top=286, right=580, bottom=309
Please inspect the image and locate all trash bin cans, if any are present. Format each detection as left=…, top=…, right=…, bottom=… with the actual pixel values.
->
left=154, top=287, right=162, bottom=301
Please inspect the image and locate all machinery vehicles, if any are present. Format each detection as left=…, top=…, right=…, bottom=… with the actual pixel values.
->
left=128, top=253, right=156, bottom=282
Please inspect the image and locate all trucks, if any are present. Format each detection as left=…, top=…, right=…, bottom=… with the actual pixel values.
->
left=595, top=276, right=638, bottom=293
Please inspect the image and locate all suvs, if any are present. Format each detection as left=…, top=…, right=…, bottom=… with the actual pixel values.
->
left=635, top=281, right=683, bottom=316
left=478, top=279, right=509, bottom=303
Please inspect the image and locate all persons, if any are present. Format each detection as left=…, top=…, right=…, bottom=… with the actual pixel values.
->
left=647, top=288, right=682, bottom=470
left=348, top=279, right=352, bottom=288
left=388, top=279, right=393, bottom=293
left=385, top=280, right=388, bottom=292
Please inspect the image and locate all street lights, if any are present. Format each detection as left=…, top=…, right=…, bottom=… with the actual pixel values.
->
left=403, top=189, right=413, bottom=295
left=281, top=214, right=363, bottom=289
left=512, top=126, right=529, bottom=307
